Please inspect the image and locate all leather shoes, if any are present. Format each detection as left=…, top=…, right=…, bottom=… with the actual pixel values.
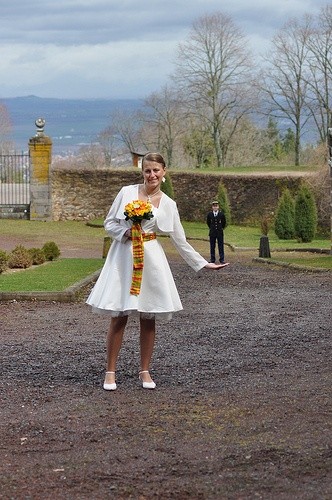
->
left=208, top=261, right=215, bottom=263
left=220, top=260, right=225, bottom=264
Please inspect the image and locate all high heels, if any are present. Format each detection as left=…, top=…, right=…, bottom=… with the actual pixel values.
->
left=138, top=371, right=156, bottom=389
left=103, top=371, right=117, bottom=390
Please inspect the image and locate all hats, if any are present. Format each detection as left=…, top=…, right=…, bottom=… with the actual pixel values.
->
left=212, top=201, right=219, bottom=204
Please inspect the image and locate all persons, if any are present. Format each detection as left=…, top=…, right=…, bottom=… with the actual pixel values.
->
left=205, top=200, right=227, bottom=264
left=84, top=152, right=231, bottom=392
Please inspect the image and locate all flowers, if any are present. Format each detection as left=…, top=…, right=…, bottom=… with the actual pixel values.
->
left=126, top=198, right=154, bottom=234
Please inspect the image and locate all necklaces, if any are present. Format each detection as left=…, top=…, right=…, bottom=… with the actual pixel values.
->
left=143, top=183, right=160, bottom=204
